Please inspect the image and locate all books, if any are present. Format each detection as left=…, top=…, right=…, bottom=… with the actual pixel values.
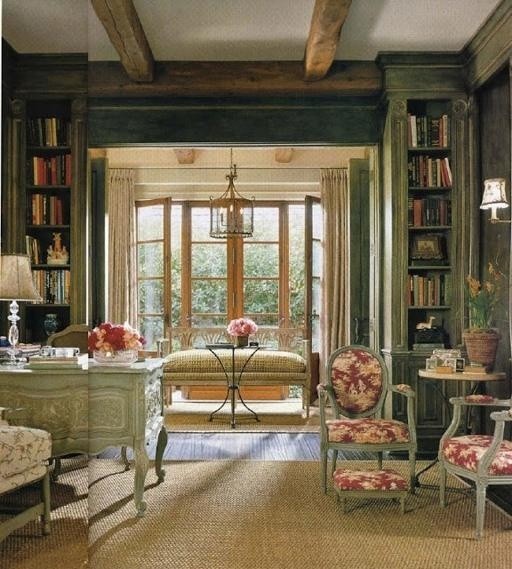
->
left=406, top=109, right=454, bottom=306
left=20, top=115, right=74, bottom=305
left=461, top=372, right=490, bottom=375
left=1, top=335, right=89, bottom=370
left=432, top=348, right=461, bottom=359
left=464, top=365, right=489, bottom=373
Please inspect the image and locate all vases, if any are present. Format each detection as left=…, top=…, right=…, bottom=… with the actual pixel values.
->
left=233, top=335, right=248, bottom=346
left=462, top=332, right=502, bottom=370
left=92, top=348, right=139, bottom=367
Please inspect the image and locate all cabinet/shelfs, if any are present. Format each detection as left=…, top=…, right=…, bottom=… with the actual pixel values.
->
left=408, top=98, right=453, bottom=349
left=26, top=99, right=71, bottom=346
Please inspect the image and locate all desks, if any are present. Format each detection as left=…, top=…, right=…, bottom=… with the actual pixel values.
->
left=0, top=359, right=167, bottom=517
left=416, top=368, right=506, bottom=487
left=194, top=345, right=272, bottom=428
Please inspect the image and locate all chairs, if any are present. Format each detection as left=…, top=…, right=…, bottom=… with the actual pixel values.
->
left=0, top=406, right=52, bottom=542
left=316, top=344, right=418, bottom=493
left=437, top=395, right=512, bottom=540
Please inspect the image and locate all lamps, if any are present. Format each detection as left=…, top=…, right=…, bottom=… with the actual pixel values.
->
left=210, top=147, right=255, bottom=239
left=479, top=178, right=511, bottom=224
left=1, top=254, right=43, bottom=364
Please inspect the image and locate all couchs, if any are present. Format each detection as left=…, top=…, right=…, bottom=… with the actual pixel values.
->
left=156, top=328, right=311, bottom=418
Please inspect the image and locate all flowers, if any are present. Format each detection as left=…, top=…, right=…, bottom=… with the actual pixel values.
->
left=227, top=318, right=258, bottom=337
left=456, top=235, right=509, bottom=329
left=88, top=321, right=146, bottom=357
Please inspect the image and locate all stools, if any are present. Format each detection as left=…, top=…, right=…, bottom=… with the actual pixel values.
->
left=332, top=468, right=408, bottom=515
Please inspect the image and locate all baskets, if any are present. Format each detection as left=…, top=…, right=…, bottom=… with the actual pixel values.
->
left=463, top=332, right=500, bottom=364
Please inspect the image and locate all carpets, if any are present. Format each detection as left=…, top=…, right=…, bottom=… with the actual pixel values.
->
left=164, top=390, right=348, bottom=432
left=0, top=459, right=512, bottom=569
